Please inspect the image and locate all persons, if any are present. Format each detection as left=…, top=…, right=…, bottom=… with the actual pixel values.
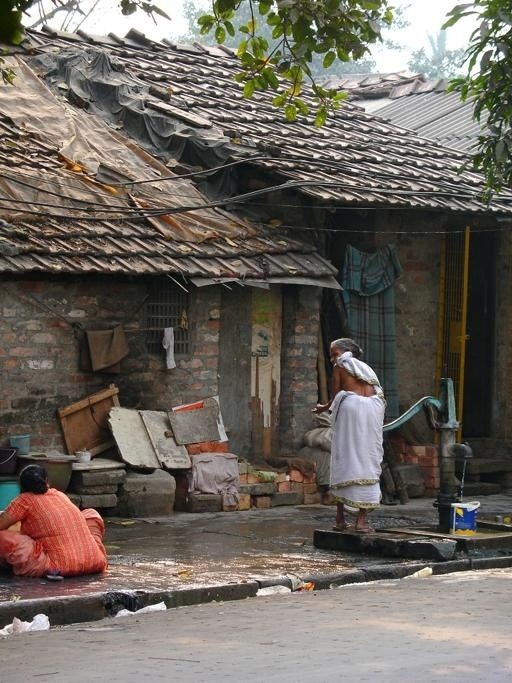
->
left=0, top=463, right=109, bottom=577
left=303, top=336, right=389, bottom=533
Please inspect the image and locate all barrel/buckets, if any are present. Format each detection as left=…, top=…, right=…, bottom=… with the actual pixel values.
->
left=10, top=433, right=30, bottom=455
left=0, top=448, right=15, bottom=475
left=450, top=500, right=481, bottom=535
left=0, top=481, right=20, bottom=509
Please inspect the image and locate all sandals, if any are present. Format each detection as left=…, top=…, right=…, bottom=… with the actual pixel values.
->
left=355, top=526, right=375, bottom=533
left=333, top=523, right=352, bottom=531
